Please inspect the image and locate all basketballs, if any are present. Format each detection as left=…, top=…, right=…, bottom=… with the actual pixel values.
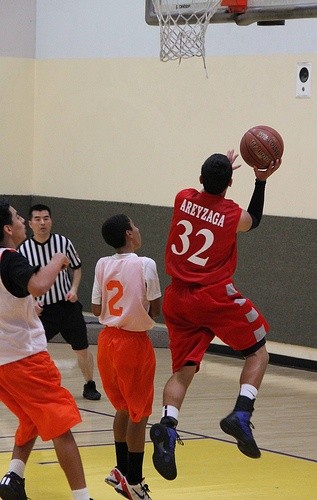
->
left=239, top=124, right=285, bottom=170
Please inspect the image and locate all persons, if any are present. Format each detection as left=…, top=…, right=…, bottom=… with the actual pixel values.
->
left=0, top=201, right=94, bottom=500
left=150, top=149, right=283, bottom=481
left=91, top=213, right=161, bottom=500
left=17, top=204, right=101, bottom=400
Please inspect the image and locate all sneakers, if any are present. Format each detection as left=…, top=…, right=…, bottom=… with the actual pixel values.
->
left=116, top=477, right=152, bottom=500
left=150, top=422, right=178, bottom=480
left=0, top=471, right=27, bottom=500
left=83, top=381, right=101, bottom=399
left=104, top=468, right=124, bottom=487
left=220, top=411, right=261, bottom=458
